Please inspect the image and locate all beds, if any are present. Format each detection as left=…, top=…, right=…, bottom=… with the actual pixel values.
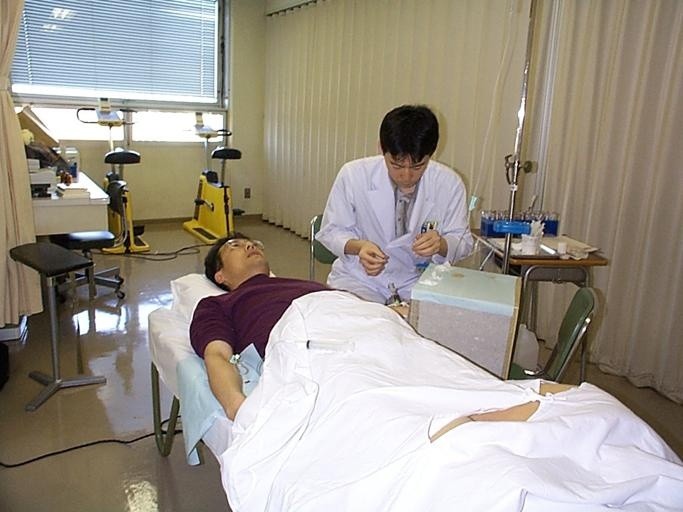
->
left=142, top=305, right=683, bottom=512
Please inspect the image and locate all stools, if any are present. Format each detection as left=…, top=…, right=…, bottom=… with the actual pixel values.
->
left=9, top=230, right=126, bottom=412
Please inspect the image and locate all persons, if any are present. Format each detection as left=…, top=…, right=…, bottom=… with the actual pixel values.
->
left=188, top=230, right=331, bottom=420
left=313, top=102, right=475, bottom=307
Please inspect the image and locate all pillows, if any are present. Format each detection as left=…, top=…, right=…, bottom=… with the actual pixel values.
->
left=164, top=269, right=277, bottom=321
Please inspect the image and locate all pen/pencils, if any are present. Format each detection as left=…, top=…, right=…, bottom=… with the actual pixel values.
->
left=420, top=221, right=437, bottom=235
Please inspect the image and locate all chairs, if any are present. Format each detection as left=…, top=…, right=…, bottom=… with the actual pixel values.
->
left=507, top=285, right=600, bottom=387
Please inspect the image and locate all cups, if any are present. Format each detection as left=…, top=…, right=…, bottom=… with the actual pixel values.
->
left=521, top=233, right=541, bottom=256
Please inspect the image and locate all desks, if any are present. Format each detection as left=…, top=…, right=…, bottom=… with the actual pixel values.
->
left=469, top=225, right=610, bottom=384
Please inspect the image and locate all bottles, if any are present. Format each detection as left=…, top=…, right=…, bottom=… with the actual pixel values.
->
left=557, top=233, right=568, bottom=256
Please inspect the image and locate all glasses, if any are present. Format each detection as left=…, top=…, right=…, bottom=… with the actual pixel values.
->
left=221, top=240, right=264, bottom=249
